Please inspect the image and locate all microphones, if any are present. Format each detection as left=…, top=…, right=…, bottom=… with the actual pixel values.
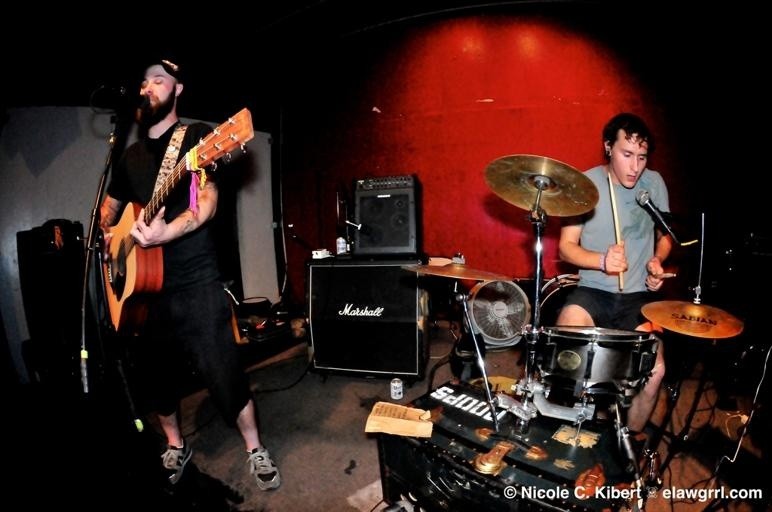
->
left=634, top=188, right=683, bottom=250
left=95, top=83, right=151, bottom=111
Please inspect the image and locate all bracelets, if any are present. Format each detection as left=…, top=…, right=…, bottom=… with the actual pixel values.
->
left=600, top=254, right=607, bottom=273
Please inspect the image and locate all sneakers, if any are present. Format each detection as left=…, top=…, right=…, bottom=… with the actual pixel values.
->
left=246, top=446, right=281, bottom=491
left=160, top=438, right=193, bottom=485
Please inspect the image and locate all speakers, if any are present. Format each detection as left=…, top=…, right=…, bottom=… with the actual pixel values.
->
left=353, top=172, right=423, bottom=256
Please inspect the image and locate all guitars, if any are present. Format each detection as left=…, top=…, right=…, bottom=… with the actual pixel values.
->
left=95, top=106, right=254, bottom=334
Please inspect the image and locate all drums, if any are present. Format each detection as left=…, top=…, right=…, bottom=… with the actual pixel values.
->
left=537, top=324, right=659, bottom=394
left=540, top=273, right=582, bottom=326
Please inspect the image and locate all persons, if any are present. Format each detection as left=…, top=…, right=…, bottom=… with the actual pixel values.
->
left=95, top=59, right=281, bottom=494
left=553, top=111, right=674, bottom=432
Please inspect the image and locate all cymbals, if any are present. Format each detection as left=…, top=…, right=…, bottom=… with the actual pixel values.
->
left=399, top=263, right=515, bottom=282
left=486, top=154, right=599, bottom=217
left=641, top=300, right=745, bottom=339
